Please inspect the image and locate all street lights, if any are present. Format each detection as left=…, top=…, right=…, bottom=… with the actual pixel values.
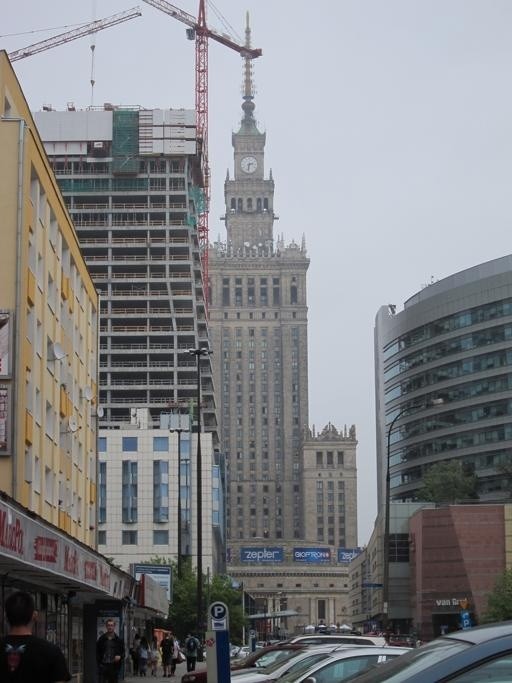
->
left=169, top=427, right=190, bottom=579
left=380, top=398, right=445, bottom=632
left=238, top=580, right=248, bottom=646
left=184, top=346, right=209, bottom=662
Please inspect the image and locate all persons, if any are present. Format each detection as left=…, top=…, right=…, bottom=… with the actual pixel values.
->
left=95, top=619, right=126, bottom=683
left=130, top=631, right=201, bottom=678
left=0, top=591, right=73, bottom=683
left=318, top=619, right=326, bottom=626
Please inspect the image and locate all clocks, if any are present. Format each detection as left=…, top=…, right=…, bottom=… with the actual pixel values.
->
left=240, top=155, right=259, bottom=174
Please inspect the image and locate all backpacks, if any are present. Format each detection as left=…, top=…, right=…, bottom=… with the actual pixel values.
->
left=188, top=638, right=196, bottom=651
